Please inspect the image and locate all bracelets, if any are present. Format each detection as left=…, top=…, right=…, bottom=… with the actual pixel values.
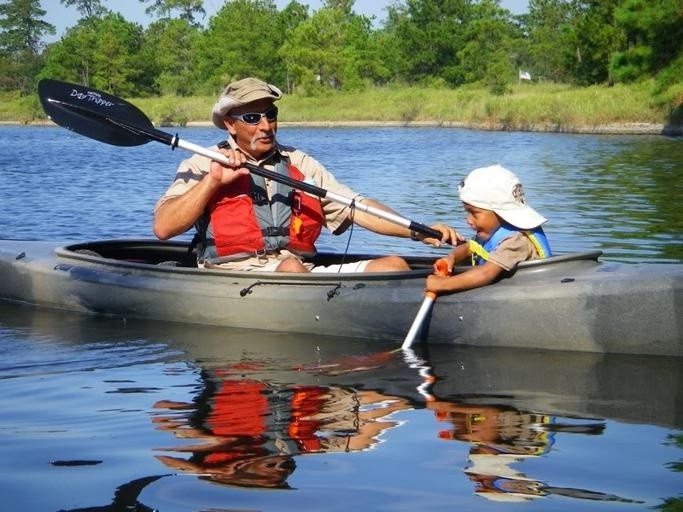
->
left=411, top=229, right=420, bottom=242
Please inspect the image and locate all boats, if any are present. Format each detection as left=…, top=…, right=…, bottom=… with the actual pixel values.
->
left=1, top=240, right=683, bottom=361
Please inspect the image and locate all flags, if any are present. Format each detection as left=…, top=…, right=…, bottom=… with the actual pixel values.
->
left=519, top=70, right=530, bottom=80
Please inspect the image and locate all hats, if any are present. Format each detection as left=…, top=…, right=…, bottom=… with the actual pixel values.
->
left=458, top=164, right=549, bottom=230
left=211, top=77, right=283, bottom=131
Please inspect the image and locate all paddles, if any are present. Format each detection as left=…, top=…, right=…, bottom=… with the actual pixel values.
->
left=37, top=79, right=467, bottom=245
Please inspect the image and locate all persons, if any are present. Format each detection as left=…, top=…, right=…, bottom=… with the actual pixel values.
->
left=147, top=359, right=413, bottom=492
left=429, top=398, right=557, bottom=501
left=152, top=73, right=465, bottom=274
left=424, top=163, right=553, bottom=292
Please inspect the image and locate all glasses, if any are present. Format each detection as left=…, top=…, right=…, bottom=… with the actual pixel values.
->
left=230, top=106, right=279, bottom=125
